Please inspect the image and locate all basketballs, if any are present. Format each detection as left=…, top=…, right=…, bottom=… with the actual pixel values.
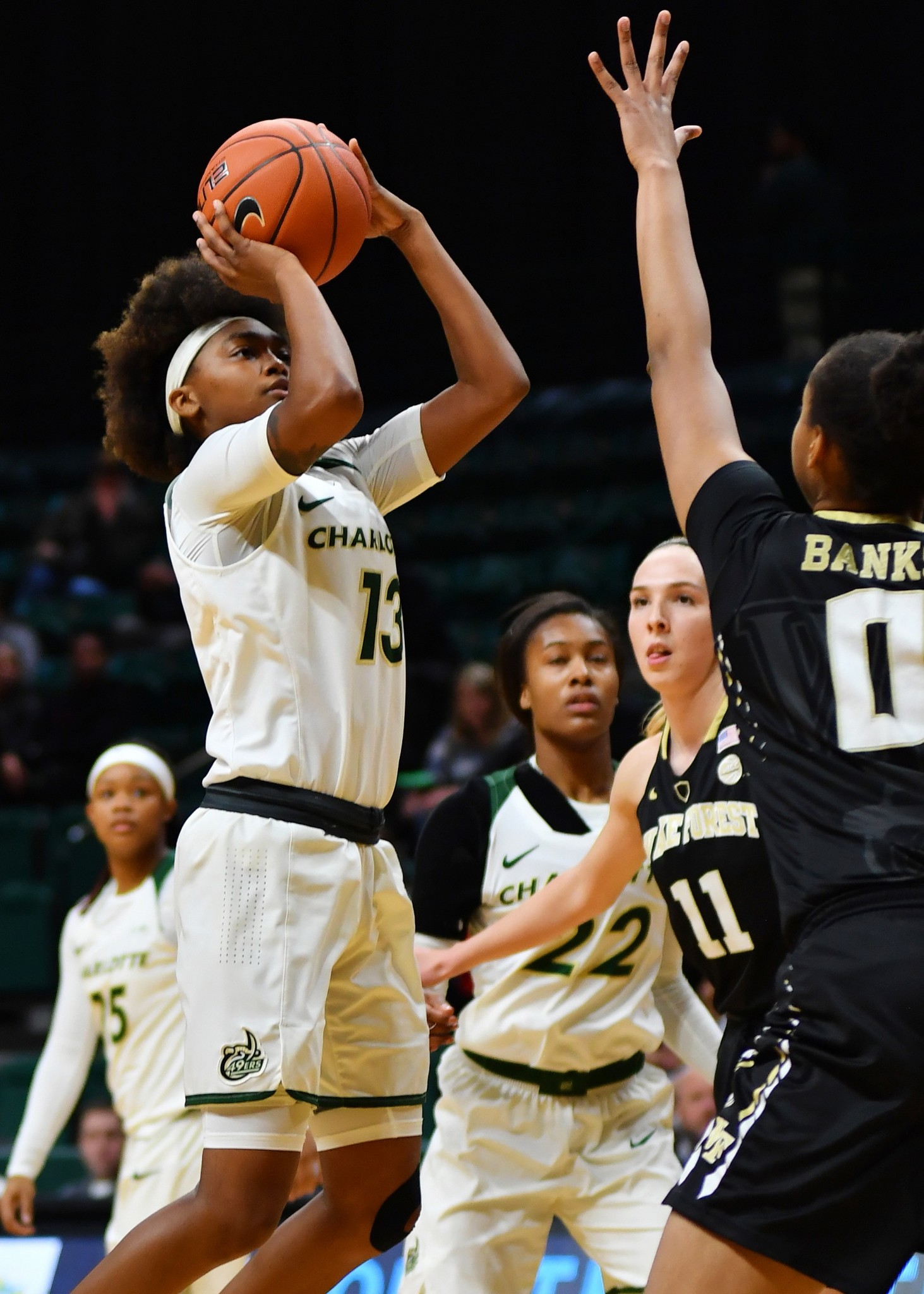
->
left=196, top=116, right=373, bottom=288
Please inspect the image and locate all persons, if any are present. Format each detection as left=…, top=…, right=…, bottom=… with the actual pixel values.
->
left=589, top=10, right=924, bottom=1293
left=382, top=660, right=530, bottom=820
left=416, top=534, right=790, bottom=1116
left=0, top=477, right=208, bottom=796
left=0, top=733, right=255, bottom=1294
left=71, top=118, right=532, bottom=1294
left=390, top=591, right=722, bottom=1294
